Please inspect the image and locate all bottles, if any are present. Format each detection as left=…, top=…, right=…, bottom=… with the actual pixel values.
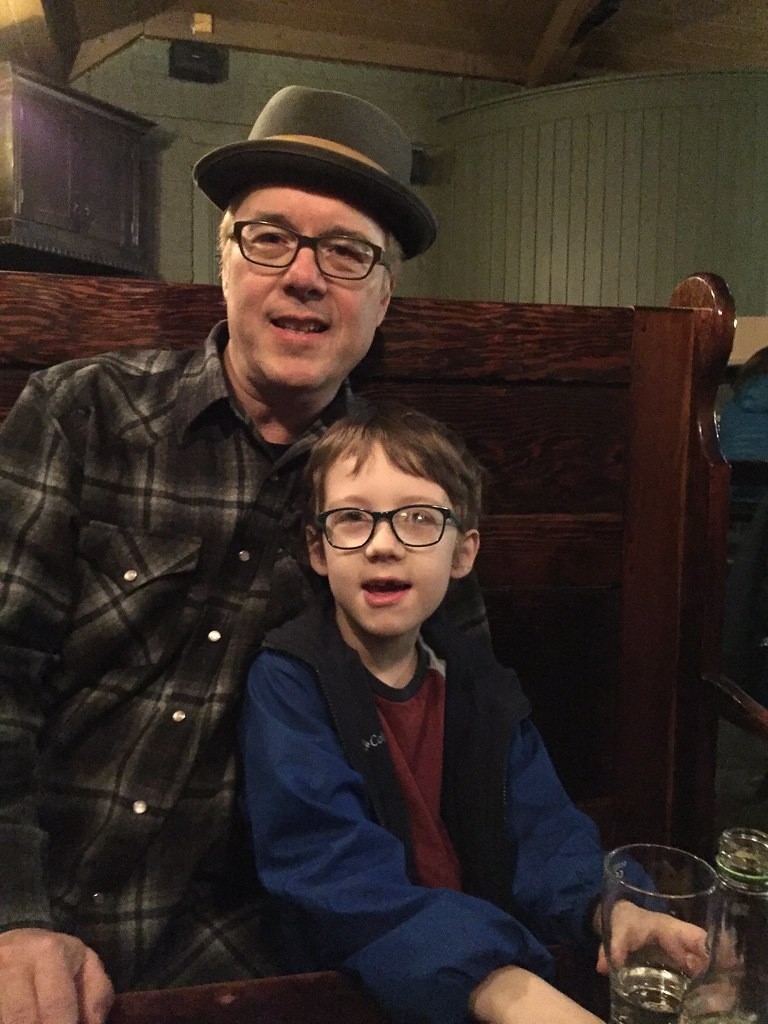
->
left=677, top=827, right=768, bottom=1024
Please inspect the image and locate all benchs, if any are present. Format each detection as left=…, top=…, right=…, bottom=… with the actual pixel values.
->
left=1, top=272, right=764, bottom=961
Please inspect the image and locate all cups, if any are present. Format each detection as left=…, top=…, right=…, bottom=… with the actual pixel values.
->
left=602, top=844, right=718, bottom=1024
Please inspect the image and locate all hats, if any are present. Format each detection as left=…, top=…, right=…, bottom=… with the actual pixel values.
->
left=191, top=84, right=439, bottom=262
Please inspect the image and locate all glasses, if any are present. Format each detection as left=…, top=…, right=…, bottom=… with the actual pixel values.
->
left=226, top=220, right=390, bottom=280
left=319, top=503, right=462, bottom=550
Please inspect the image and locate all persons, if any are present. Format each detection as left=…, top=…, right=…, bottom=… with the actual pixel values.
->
left=0, top=85, right=440, bottom=1024
left=245, top=399, right=714, bottom=1024
left=719, top=346, right=768, bottom=461
